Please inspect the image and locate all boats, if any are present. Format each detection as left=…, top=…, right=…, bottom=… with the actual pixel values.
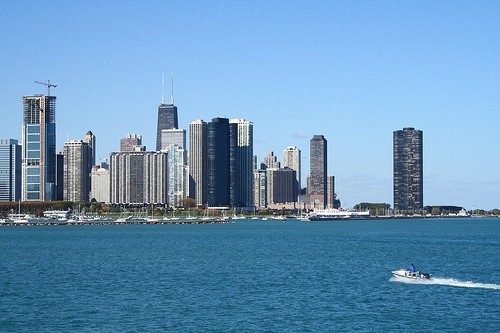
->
left=390, top=269, right=431, bottom=281
left=0, top=207, right=499, bottom=225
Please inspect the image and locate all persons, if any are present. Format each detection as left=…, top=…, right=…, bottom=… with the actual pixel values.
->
left=409, top=264, right=416, bottom=273
